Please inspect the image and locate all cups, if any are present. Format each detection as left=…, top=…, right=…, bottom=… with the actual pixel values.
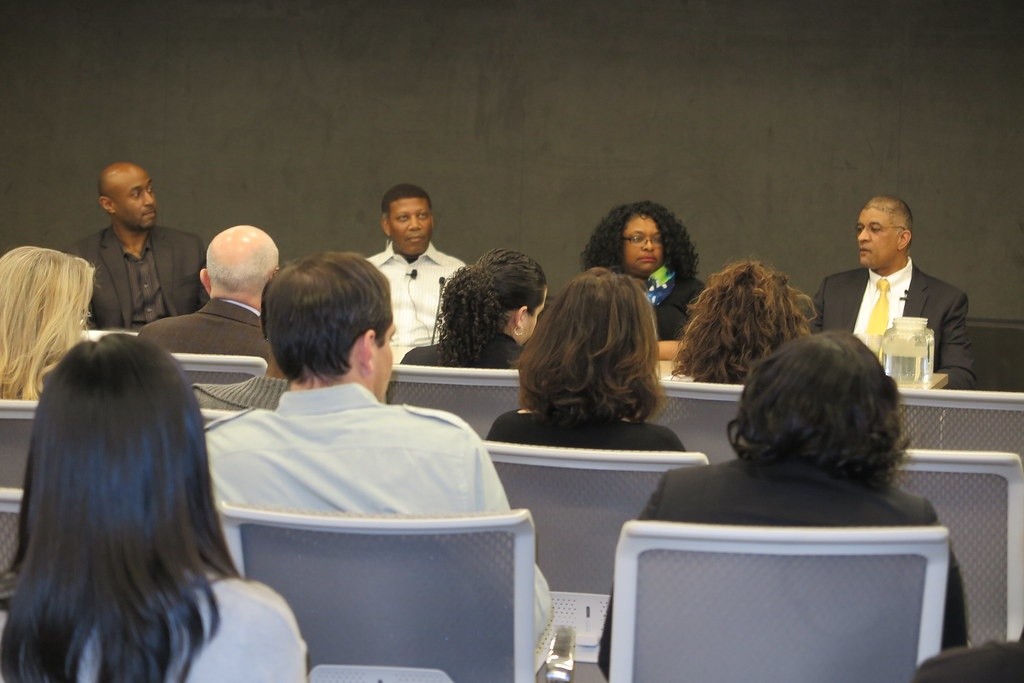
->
left=852, top=334, right=882, bottom=360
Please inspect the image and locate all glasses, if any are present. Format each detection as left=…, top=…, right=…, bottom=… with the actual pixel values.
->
left=49, top=303, right=85, bottom=323
left=621, top=233, right=663, bottom=246
left=855, top=221, right=906, bottom=234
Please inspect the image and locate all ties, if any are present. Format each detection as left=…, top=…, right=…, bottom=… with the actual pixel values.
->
left=866, top=278, right=888, bottom=366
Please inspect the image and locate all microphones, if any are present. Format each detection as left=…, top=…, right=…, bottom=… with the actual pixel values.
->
left=905, top=289, right=909, bottom=295
left=650, top=279, right=658, bottom=309
left=410, top=269, right=417, bottom=280
left=431, top=277, right=445, bottom=345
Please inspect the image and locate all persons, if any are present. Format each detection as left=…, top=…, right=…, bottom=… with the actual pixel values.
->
left=138, top=225, right=279, bottom=365
left=401, top=248, right=548, bottom=369
left=0, top=246, right=96, bottom=401
left=0, top=331, right=308, bottom=683
left=365, top=184, right=468, bottom=365
left=61, top=162, right=210, bottom=331
left=191, top=252, right=552, bottom=652
left=580, top=200, right=706, bottom=341
left=808, top=195, right=978, bottom=390
left=484, top=267, right=687, bottom=452
left=597, top=330, right=966, bottom=683
left=670, top=260, right=817, bottom=384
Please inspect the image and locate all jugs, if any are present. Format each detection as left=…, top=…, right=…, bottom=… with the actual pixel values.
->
left=882, top=316, right=935, bottom=389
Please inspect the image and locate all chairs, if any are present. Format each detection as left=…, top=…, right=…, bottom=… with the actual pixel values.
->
left=899, top=386, right=1024, bottom=461
left=215, top=498, right=537, bottom=683
left=966, top=318, right=1024, bottom=392
left=484, top=439, right=710, bottom=663
left=175, top=351, right=267, bottom=384
left=608, top=521, right=949, bottom=683
left=645, top=380, right=743, bottom=465
left=386, top=364, right=525, bottom=437
left=891, top=450, right=1024, bottom=644
